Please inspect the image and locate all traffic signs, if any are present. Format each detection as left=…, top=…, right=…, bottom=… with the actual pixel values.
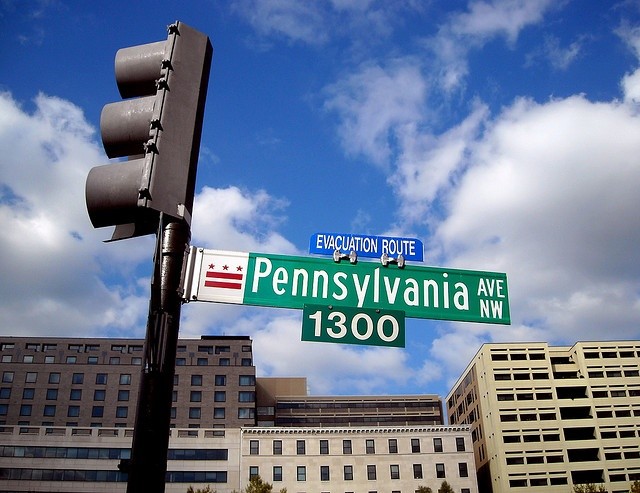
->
left=183, top=246, right=511, bottom=346
left=309, top=231, right=423, bottom=262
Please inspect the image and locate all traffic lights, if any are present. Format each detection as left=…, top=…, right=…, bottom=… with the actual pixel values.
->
left=86, top=19, right=213, bottom=242
